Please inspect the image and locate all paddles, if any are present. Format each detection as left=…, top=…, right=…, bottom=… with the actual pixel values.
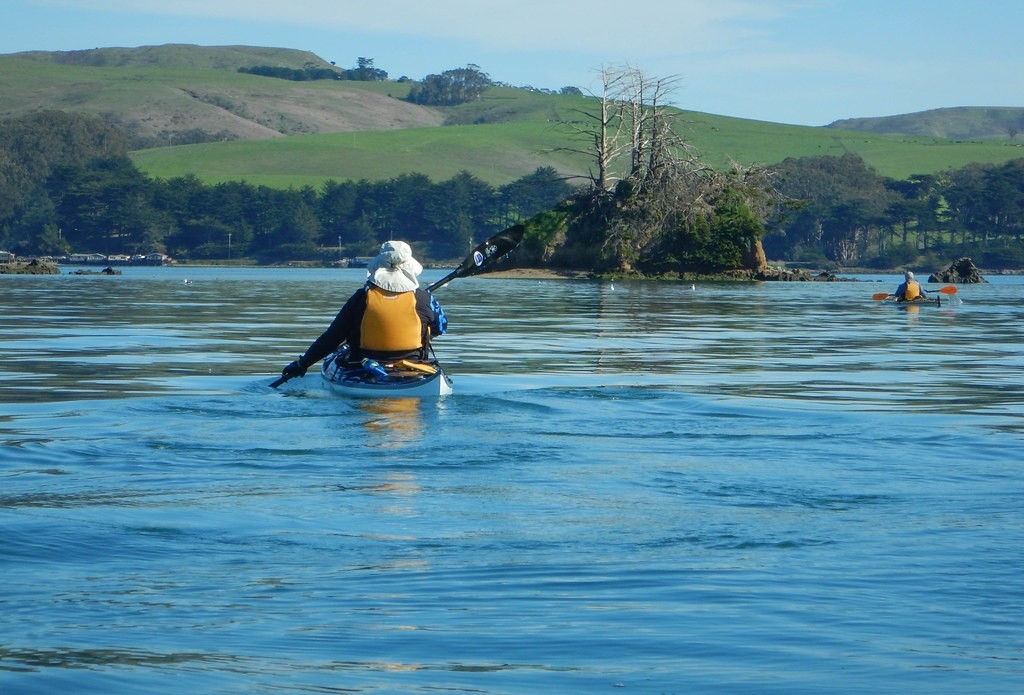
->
left=267, top=221, right=528, bottom=388
left=872, top=285, right=957, bottom=300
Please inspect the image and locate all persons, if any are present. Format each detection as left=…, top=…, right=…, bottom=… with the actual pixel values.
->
left=281, top=240, right=448, bottom=378
left=895, top=271, right=927, bottom=301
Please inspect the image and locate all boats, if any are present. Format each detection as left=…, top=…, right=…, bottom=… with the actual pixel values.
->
left=320, top=344, right=453, bottom=398
left=894, top=295, right=940, bottom=307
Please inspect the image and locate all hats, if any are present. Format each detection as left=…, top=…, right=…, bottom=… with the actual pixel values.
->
left=905, top=272, right=914, bottom=280
left=366, top=240, right=424, bottom=292
